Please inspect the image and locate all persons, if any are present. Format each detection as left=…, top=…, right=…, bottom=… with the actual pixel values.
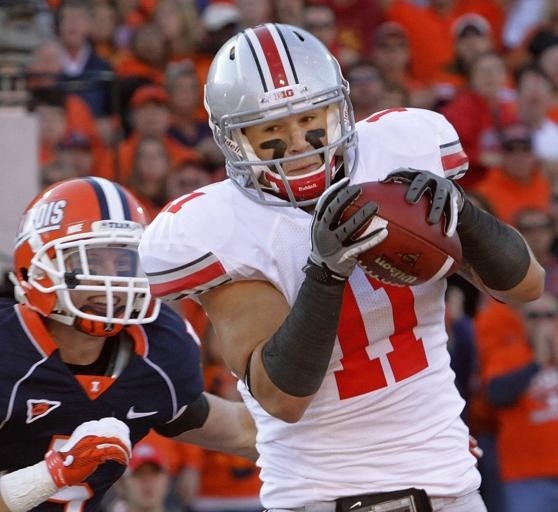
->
left=133, top=21, right=547, bottom=512
left=1, top=172, right=258, bottom=508
left=105, top=442, right=194, bottom=512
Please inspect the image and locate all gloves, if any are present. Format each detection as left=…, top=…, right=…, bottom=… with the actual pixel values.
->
left=379, top=165, right=470, bottom=238
left=43, top=415, right=135, bottom=490
left=300, top=176, right=389, bottom=289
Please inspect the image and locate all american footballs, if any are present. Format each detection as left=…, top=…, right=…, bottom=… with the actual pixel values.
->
left=338, top=182, right=462, bottom=286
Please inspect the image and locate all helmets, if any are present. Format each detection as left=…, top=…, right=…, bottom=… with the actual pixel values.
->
left=8, top=173, right=157, bottom=323
left=202, top=19, right=358, bottom=187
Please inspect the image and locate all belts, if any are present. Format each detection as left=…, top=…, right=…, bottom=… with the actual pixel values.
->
left=293, top=487, right=460, bottom=512
